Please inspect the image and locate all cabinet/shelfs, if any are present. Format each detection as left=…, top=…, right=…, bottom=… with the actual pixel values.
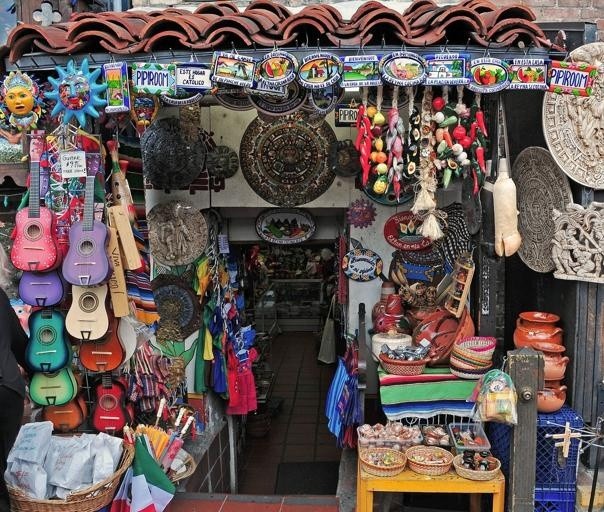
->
left=255, top=278, right=324, bottom=332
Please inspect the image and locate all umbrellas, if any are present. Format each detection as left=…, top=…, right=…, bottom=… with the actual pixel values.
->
left=137, top=398, right=171, bottom=464
left=161, top=414, right=195, bottom=474
left=157, top=405, right=186, bottom=466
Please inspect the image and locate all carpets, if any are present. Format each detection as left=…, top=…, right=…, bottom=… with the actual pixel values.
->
left=275, top=460, right=340, bottom=495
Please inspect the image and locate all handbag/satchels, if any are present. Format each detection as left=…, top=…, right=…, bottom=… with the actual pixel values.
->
left=324, top=341, right=363, bottom=449
left=317, top=294, right=336, bottom=365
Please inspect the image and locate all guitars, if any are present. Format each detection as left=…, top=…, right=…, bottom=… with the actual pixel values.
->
left=10, top=139, right=132, bottom=435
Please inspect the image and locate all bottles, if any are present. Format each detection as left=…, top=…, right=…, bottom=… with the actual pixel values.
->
left=514, top=310, right=568, bottom=412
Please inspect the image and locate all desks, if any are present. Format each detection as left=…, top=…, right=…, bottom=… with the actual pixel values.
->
left=356, top=438, right=505, bottom=512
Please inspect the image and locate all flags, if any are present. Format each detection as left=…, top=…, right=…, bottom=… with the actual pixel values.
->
left=109, top=428, right=176, bottom=512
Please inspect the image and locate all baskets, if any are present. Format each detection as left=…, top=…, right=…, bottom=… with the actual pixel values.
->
left=449, top=422, right=501, bottom=481
left=405, top=445, right=454, bottom=476
left=449, top=335, right=497, bottom=379
left=5, top=433, right=135, bottom=512
left=485, top=404, right=583, bottom=512
left=380, top=350, right=431, bottom=376
left=359, top=448, right=407, bottom=477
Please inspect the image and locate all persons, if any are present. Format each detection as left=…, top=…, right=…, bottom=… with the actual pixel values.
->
left=550, top=49, right=604, bottom=279
left=0, top=287, right=39, bottom=511
